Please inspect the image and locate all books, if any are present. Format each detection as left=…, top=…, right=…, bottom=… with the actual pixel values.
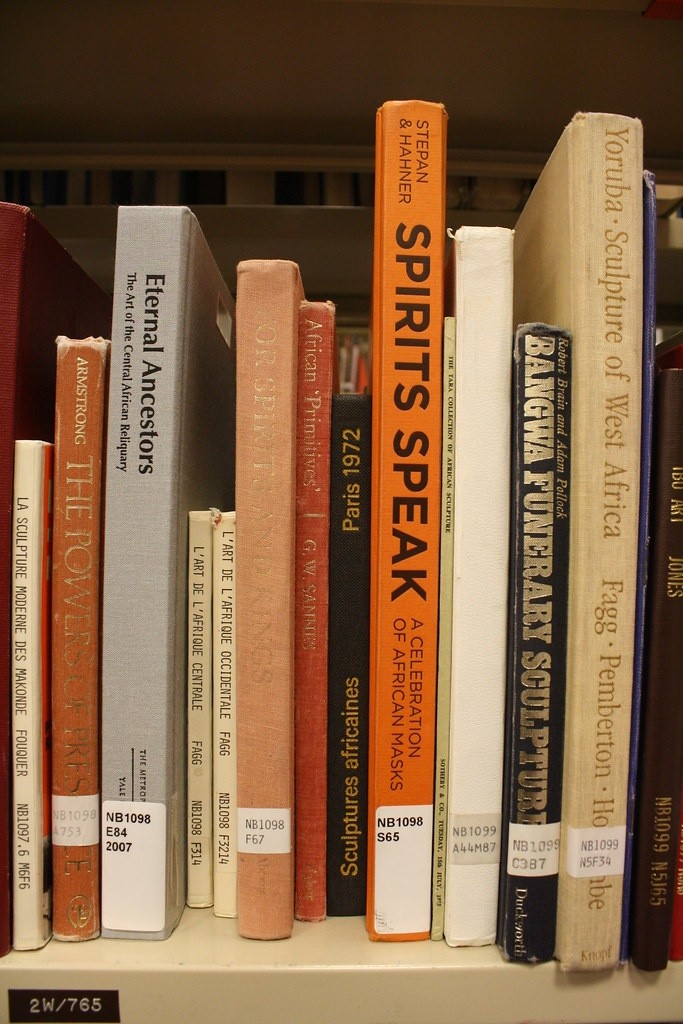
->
left=12, top=437, right=56, bottom=954
left=235, top=258, right=306, bottom=940
left=102, top=203, right=235, bottom=943
left=327, top=318, right=373, bottom=919
left=516, top=110, right=683, bottom=971
left=51, top=332, right=110, bottom=946
left=444, top=225, right=514, bottom=950
left=0, top=201, right=112, bottom=957
left=366, top=96, right=457, bottom=945
left=293, top=299, right=335, bottom=923
left=186, top=510, right=236, bottom=918
left=496, top=320, right=573, bottom=966
